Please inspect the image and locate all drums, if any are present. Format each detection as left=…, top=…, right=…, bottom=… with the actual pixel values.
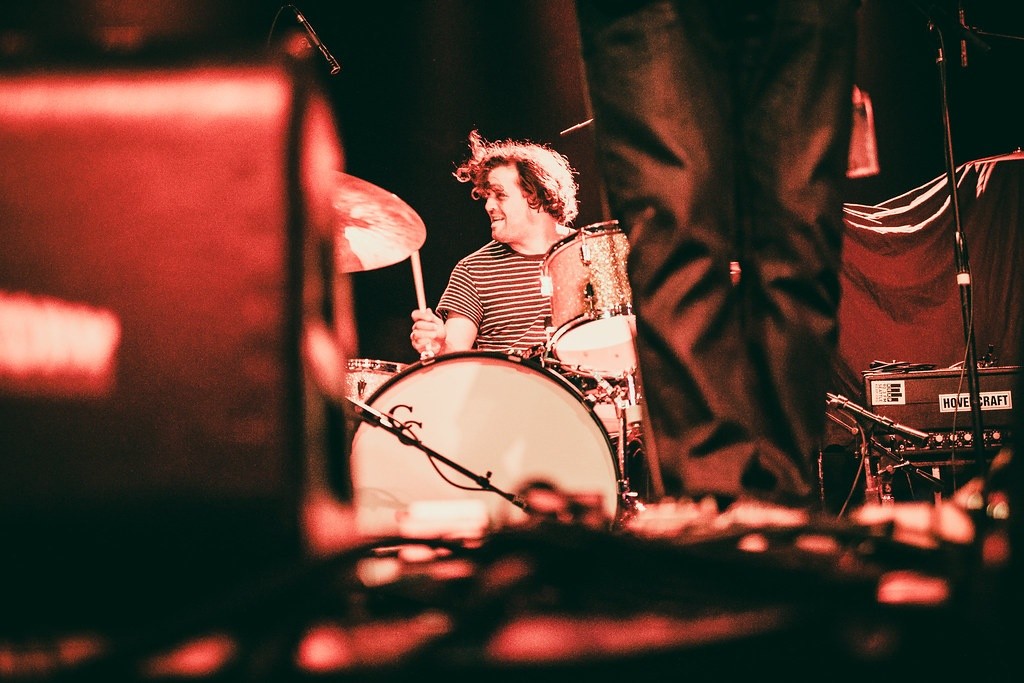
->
left=539, top=219, right=638, bottom=378
left=349, top=350, right=621, bottom=522
left=345, top=357, right=411, bottom=434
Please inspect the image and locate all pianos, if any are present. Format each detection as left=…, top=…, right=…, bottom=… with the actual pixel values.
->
left=863, top=363, right=1024, bottom=431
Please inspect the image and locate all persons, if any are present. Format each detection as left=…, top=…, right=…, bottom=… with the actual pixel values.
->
left=410, top=131, right=580, bottom=358
left=575, top=0, right=860, bottom=514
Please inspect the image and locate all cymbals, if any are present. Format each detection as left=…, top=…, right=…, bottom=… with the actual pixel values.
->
left=332, top=170, right=427, bottom=275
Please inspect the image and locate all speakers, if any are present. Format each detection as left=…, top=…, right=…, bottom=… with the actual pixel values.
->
left=1, top=70, right=362, bottom=641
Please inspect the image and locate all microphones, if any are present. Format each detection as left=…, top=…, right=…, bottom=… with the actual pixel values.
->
left=289, top=4, right=340, bottom=75
left=959, top=8, right=967, bottom=67
